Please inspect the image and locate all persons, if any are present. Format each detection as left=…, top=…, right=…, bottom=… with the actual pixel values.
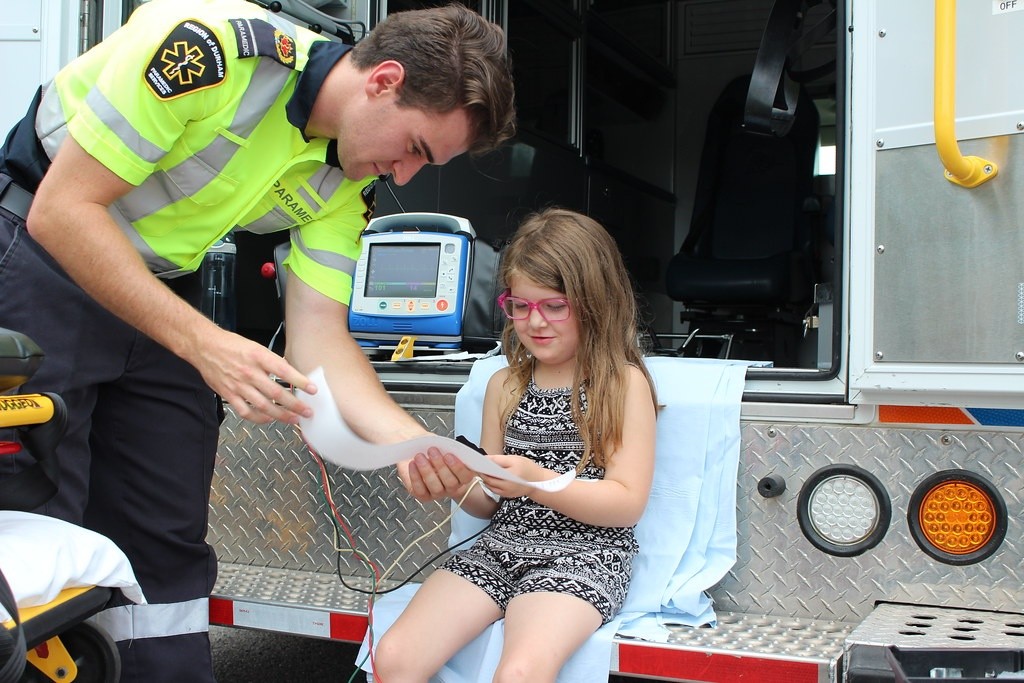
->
left=373, top=209, right=666, bottom=683
left=0, top=0, right=518, bottom=683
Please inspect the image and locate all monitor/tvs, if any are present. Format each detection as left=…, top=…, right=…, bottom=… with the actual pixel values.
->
left=351, top=229, right=470, bottom=354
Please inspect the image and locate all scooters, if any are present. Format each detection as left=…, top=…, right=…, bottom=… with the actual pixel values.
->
left=0, top=389, right=123, bottom=683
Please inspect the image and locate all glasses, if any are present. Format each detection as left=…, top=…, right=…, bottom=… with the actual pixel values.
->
left=497, top=288, right=572, bottom=322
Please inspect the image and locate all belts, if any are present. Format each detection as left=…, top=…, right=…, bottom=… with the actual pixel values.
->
left=0, top=172, right=35, bottom=221
